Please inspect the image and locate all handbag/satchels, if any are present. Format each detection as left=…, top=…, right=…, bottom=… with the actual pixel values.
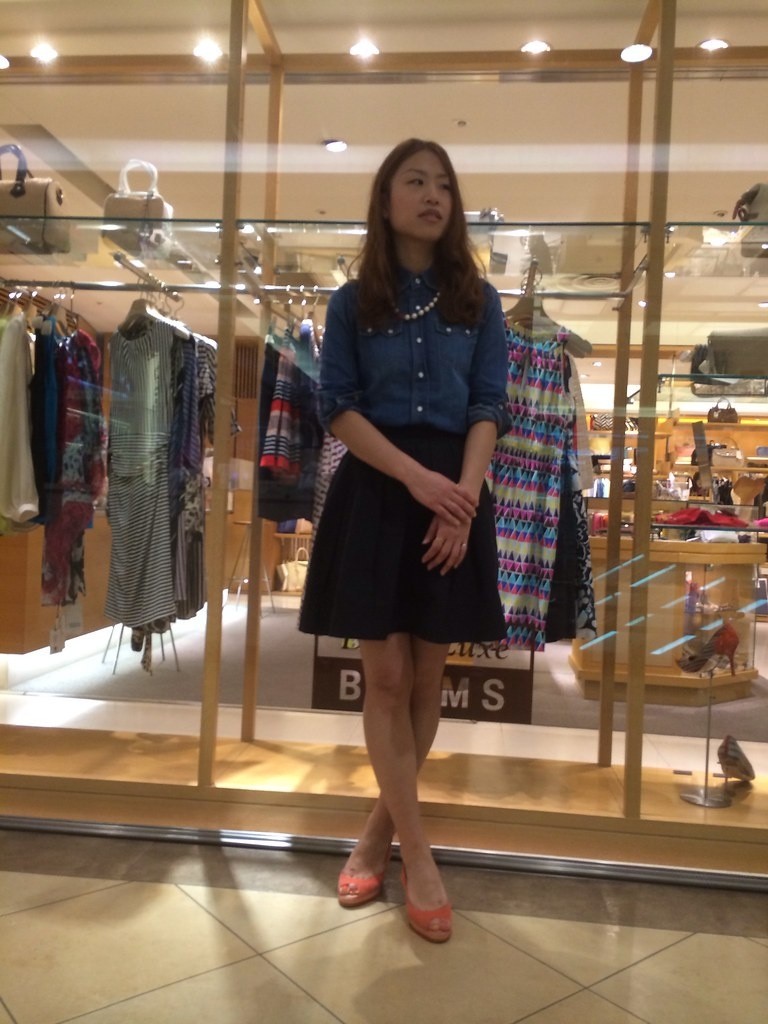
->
left=277, top=548, right=309, bottom=593
left=712, top=436, right=744, bottom=468
left=690, top=441, right=726, bottom=466
left=708, top=397, right=738, bottom=423
left=0, top=144, right=70, bottom=255
left=100, top=160, right=167, bottom=250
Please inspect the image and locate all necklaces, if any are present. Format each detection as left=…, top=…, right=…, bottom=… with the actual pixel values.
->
left=384, top=282, right=441, bottom=320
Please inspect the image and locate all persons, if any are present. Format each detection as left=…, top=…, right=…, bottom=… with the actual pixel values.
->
left=298, top=137, right=514, bottom=948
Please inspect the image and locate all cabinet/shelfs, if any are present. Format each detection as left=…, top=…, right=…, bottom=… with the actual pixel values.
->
left=0, top=1, right=768, bottom=892
left=584, top=409, right=767, bottom=543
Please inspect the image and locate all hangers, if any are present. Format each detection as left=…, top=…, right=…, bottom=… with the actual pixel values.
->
left=0, top=276, right=218, bottom=340
left=502, top=266, right=593, bottom=358
left=266, top=284, right=322, bottom=351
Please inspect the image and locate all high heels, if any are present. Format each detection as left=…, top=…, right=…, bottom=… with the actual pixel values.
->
left=675, top=623, right=738, bottom=677
left=717, top=735, right=756, bottom=795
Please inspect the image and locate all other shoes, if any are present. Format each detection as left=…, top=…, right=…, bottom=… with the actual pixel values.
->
left=399, top=860, right=452, bottom=941
left=338, top=839, right=392, bottom=905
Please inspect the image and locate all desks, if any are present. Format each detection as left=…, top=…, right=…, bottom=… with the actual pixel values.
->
left=222, top=520, right=276, bottom=618
